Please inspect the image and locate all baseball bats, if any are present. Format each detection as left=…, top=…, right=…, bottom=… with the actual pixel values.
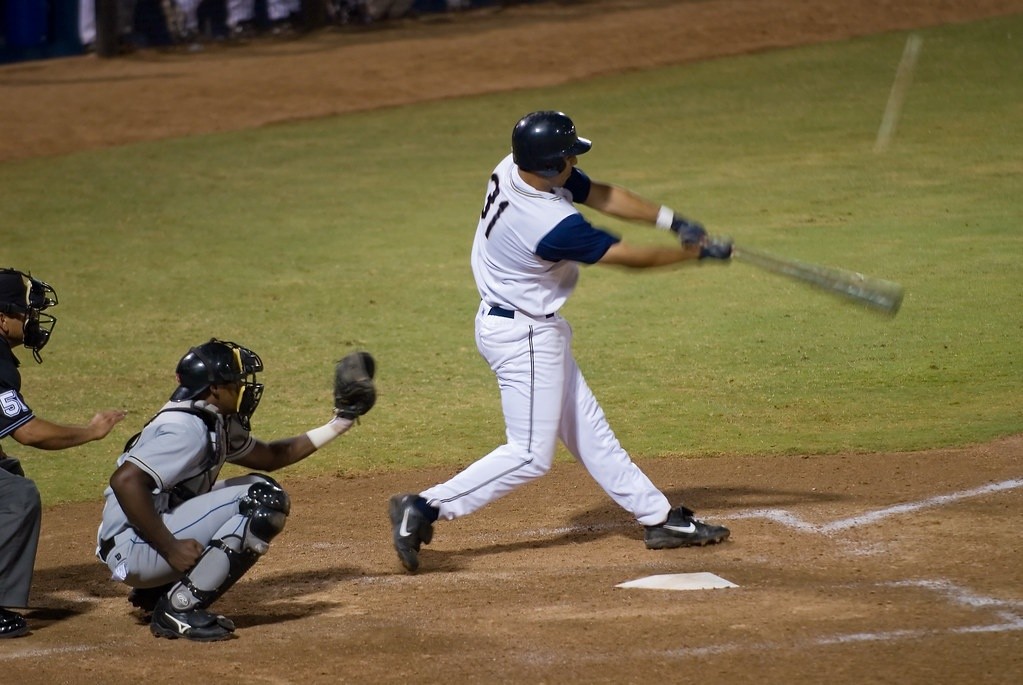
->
left=700, top=235, right=903, bottom=316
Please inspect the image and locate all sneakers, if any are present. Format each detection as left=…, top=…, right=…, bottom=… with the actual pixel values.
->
left=128, top=582, right=174, bottom=615
left=643, top=506, right=730, bottom=549
left=391, top=494, right=433, bottom=572
left=150, top=592, right=230, bottom=641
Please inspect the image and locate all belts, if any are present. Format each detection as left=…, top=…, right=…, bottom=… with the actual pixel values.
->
left=98, top=537, right=116, bottom=562
left=488, top=307, right=554, bottom=319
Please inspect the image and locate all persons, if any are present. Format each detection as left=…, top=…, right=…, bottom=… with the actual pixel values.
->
left=94, top=335, right=378, bottom=644
left=76, top=0, right=478, bottom=61
left=387, top=109, right=735, bottom=574
left=0, top=264, right=129, bottom=641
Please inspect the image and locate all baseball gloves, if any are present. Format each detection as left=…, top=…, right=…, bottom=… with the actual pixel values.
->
left=332, top=352, right=376, bottom=420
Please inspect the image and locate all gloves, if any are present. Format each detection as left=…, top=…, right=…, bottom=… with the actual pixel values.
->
left=656, top=205, right=731, bottom=260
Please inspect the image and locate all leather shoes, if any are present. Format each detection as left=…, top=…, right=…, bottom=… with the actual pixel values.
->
left=0, top=607, right=30, bottom=637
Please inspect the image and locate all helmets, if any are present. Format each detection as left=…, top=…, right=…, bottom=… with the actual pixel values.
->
left=512, top=111, right=592, bottom=176
left=169, top=343, right=251, bottom=402
left=0, top=271, right=45, bottom=313
